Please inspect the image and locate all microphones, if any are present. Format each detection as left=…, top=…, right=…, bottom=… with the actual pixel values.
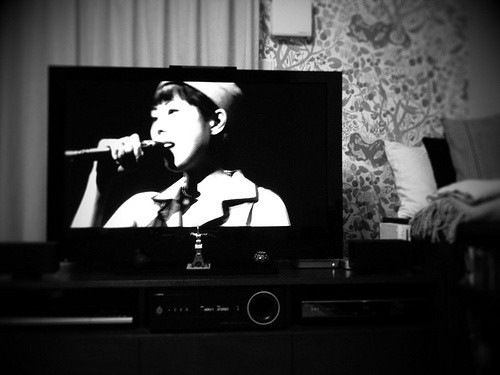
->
left=64, top=140, right=161, bottom=166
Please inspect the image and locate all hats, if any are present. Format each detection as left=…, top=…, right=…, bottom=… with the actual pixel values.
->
left=157, top=80, right=244, bottom=116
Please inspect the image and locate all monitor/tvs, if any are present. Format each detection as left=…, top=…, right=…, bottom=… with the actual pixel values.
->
left=46, top=65, right=343, bottom=252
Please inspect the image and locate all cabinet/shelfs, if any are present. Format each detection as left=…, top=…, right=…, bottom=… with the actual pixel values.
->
left=0, top=239, right=500, bottom=375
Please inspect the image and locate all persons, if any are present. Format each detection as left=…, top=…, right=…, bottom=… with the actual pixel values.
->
left=65, top=76, right=292, bottom=229
